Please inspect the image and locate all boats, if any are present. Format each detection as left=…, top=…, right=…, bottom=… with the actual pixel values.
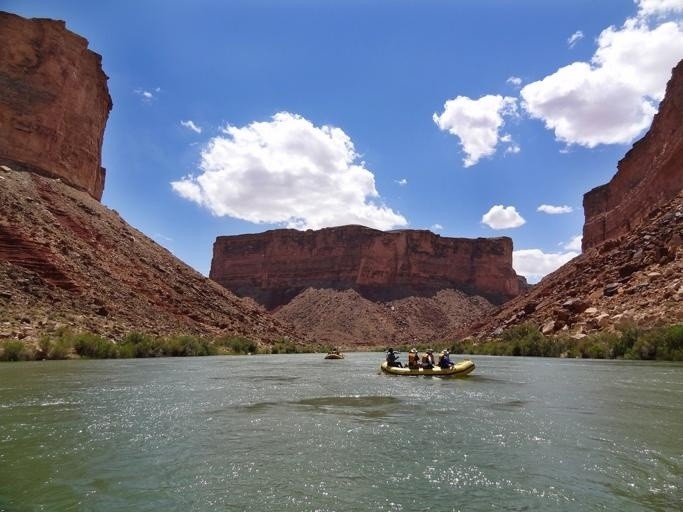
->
left=324, top=352, right=344, bottom=360
left=380, top=360, right=476, bottom=378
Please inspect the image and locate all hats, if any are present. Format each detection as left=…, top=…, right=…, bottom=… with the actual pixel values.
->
left=441, top=350, right=449, bottom=355
left=410, top=348, right=418, bottom=353
left=426, top=349, right=434, bottom=353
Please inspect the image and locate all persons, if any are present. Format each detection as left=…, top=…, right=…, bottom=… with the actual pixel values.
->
left=421, top=349, right=434, bottom=369
left=386, top=348, right=403, bottom=368
left=408, top=348, right=421, bottom=369
left=327, top=346, right=340, bottom=355
left=437, top=350, right=456, bottom=369
left=446, top=348, right=449, bottom=360
left=429, top=349, right=435, bottom=365
